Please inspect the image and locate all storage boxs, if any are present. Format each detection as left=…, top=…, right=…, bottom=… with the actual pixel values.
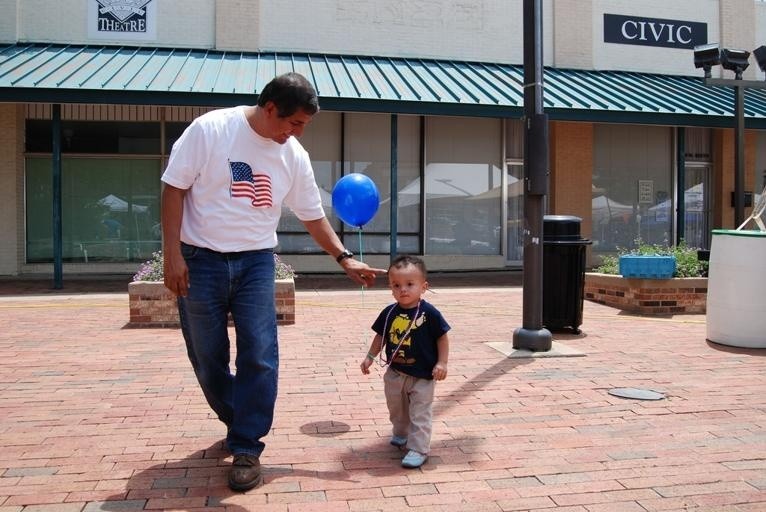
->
left=620, top=253, right=679, bottom=282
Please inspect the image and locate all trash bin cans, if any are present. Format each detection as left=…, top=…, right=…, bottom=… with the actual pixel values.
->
left=543, top=215, right=593, bottom=335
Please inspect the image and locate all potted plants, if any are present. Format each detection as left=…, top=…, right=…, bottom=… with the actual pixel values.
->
left=584, top=231, right=709, bottom=317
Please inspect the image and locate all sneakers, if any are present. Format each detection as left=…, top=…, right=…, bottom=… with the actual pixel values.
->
left=226, top=421, right=238, bottom=448
left=389, top=433, right=409, bottom=446
left=401, top=449, right=431, bottom=467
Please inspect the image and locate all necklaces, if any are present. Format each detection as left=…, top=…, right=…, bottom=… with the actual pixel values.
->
left=380, top=300, right=421, bottom=368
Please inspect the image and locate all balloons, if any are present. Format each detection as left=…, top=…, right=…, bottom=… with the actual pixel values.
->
left=332, top=172, right=381, bottom=228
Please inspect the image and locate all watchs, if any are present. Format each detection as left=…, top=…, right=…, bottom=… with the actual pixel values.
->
left=336, top=249, right=351, bottom=264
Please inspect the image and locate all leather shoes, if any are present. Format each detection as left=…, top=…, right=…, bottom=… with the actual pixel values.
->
left=227, top=453, right=263, bottom=494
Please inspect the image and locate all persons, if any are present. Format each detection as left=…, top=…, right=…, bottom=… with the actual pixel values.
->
left=361, top=253, right=451, bottom=467
left=159, top=72, right=387, bottom=490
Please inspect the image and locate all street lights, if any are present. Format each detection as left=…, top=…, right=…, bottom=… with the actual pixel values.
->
left=692, top=42, right=765, bottom=228
